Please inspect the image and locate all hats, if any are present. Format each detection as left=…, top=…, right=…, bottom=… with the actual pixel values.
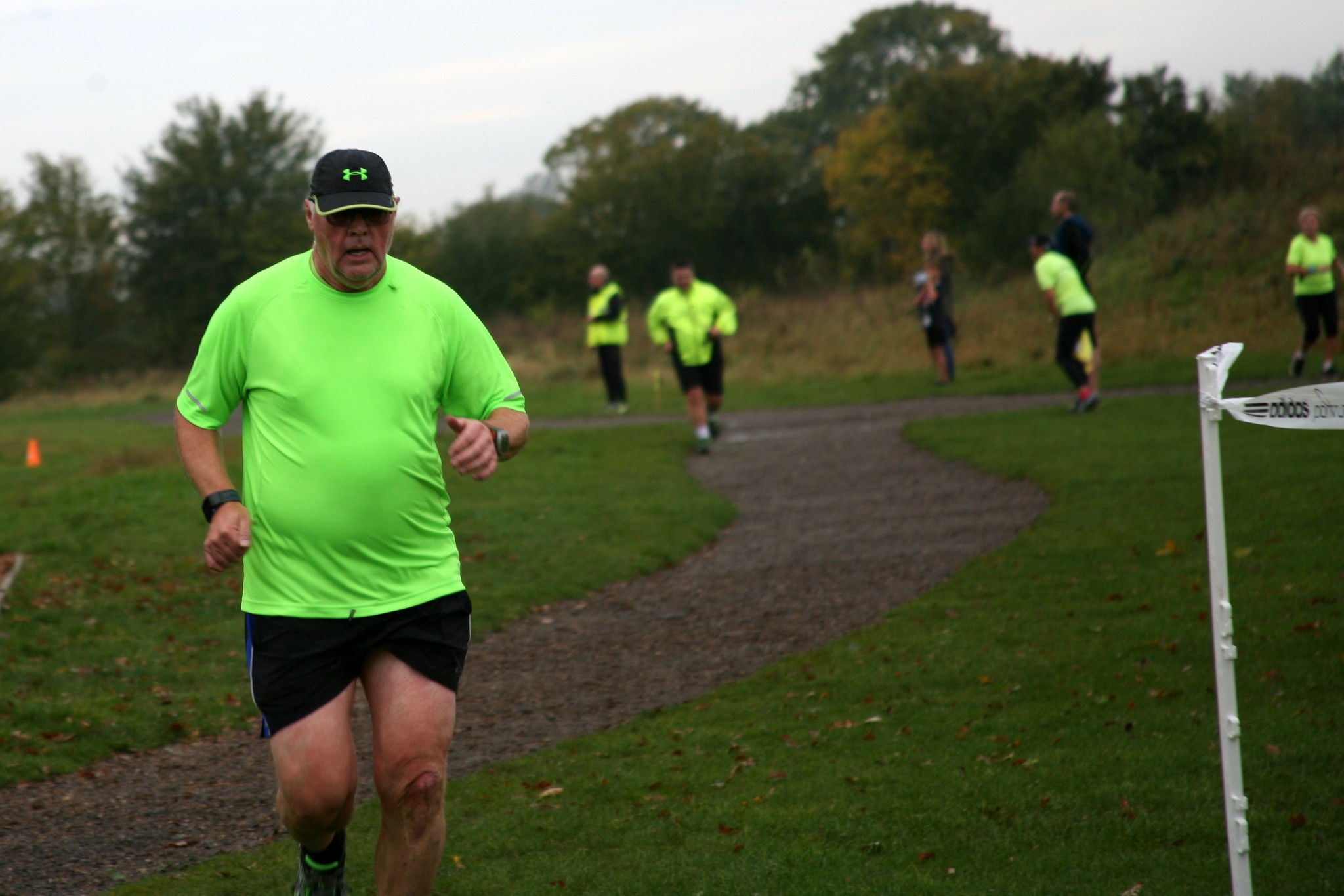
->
left=309, top=149, right=397, bottom=216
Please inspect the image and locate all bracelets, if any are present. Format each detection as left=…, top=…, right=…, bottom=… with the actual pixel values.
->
left=1309, top=267, right=1315, bottom=274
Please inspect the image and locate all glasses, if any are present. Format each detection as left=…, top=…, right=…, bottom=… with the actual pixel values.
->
left=309, top=196, right=390, bottom=225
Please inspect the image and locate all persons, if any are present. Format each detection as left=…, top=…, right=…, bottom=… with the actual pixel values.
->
left=645, top=258, right=738, bottom=453
left=585, top=264, right=630, bottom=416
left=173, top=148, right=530, bottom=896
left=1286, top=205, right=1344, bottom=379
left=913, top=230, right=957, bottom=386
left=0, top=554, right=25, bottom=591
left=1026, top=231, right=1102, bottom=414
left=1050, top=187, right=1094, bottom=291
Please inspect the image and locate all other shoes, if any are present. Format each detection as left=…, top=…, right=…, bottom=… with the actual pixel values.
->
left=1290, top=353, right=1303, bottom=377
left=696, top=438, right=708, bottom=450
left=1072, top=392, right=1096, bottom=415
left=1322, top=366, right=1337, bottom=378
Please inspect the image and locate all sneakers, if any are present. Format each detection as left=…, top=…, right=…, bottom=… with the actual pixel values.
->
left=295, top=828, right=347, bottom=896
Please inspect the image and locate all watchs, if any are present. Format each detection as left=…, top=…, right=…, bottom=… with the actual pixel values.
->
left=482, top=421, right=510, bottom=461
left=201, top=488, right=242, bottom=524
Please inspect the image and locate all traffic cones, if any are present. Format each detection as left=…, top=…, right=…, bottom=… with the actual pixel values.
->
left=26, top=438, right=41, bottom=468
